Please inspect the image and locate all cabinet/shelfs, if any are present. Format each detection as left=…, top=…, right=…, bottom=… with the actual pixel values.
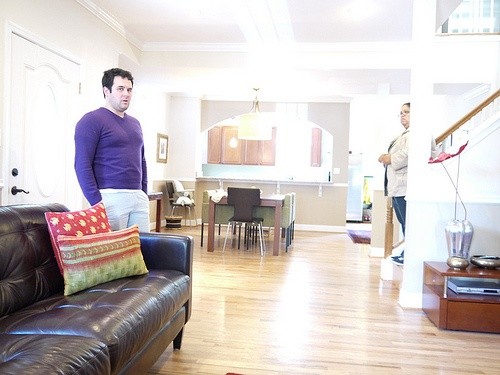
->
left=422, top=261, right=500, bottom=333
left=208, top=125, right=322, bottom=167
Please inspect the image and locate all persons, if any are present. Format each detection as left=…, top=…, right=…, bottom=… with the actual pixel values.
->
left=74, top=68, right=150, bottom=233
left=377, top=102, right=410, bottom=266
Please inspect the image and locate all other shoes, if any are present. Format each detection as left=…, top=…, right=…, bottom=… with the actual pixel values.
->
left=391, top=256, right=403, bottom=265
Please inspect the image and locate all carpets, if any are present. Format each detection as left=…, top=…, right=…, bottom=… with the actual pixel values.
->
left=347, top=229, right=371, bottom=244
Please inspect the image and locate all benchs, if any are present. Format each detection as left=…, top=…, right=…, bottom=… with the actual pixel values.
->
left=0, top=202, right=194, bottom=375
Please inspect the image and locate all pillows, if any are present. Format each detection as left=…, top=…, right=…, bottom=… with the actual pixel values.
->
left=44, top=202, right=150, bottom=296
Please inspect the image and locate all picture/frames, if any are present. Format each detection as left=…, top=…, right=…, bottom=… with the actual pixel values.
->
left=157, top=132, right=168, bottom=163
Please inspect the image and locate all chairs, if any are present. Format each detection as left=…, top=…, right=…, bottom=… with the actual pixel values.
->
left=223, top=187, right=266, bottom=257
left=166, top=179, right=197, bottom=228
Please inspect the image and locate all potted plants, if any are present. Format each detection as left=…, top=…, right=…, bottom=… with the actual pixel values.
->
left=165, top=206, right=184, bottom=230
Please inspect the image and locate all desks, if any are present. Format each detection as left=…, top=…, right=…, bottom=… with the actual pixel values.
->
left=201, top=189, right=296, bottom=257
left=207, top=195, right=286, bottom=256
left=149, top=192, right=164, bottom=232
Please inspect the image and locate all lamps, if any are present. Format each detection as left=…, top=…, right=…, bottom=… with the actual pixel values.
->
left=236, top=88, right=272, bottom=140
left=426, top=134, right=474, bottom=258
